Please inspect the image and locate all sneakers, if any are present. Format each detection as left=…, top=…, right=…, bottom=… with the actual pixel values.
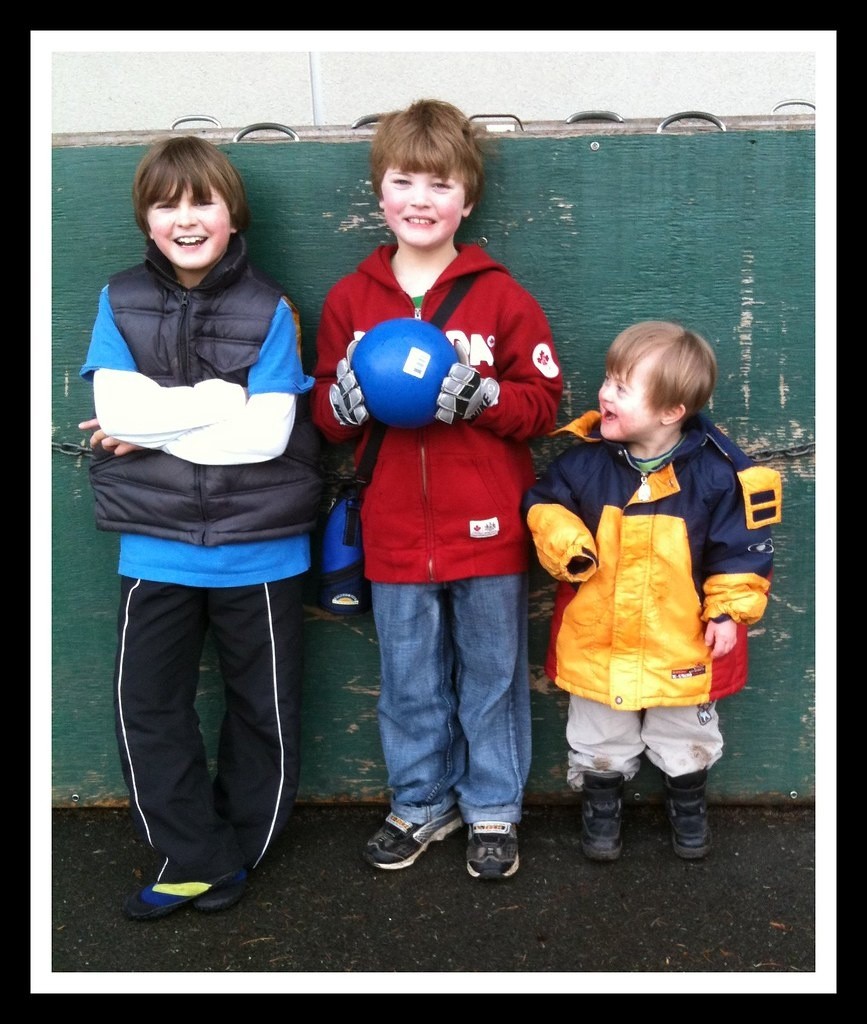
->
left=465, top=820, right=520, bottom=881
left=362, top=807, right=464, bottom=870
left=663, top=770, right=714, bottom=860
left=580, top=769, right=624, bottom=862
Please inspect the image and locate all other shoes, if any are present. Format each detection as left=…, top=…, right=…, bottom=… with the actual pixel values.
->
left=195, top=874, right=251, bottom=911
left=125, top=864, right=248, bottom=920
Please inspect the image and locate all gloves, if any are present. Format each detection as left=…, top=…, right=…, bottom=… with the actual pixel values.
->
left=434, top=338, right=487, bottom=425
left=327, top=331, right=370, bottom=426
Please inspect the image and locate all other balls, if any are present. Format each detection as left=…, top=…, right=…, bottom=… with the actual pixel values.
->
left=350, top=317, right=461, bottom=429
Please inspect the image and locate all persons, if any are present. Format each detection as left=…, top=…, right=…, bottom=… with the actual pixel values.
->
left=523, top=321, right=782, bottom=861
left=311, top=99, right=565, bottom=879
left=77, top=136, right=323, bottom=925
